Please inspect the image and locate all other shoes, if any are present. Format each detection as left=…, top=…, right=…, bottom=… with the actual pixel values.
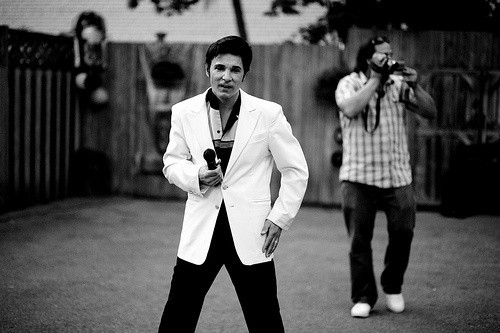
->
left=386, top=293, right=405, bottom=312
left=350, top=301, right=371, bottom=318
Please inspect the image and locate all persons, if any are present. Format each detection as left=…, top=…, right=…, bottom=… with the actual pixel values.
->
left=333, top=34, right=440, bottom=318
left=132, top=41, right=196, bottom=177
left=157, top=35, right=309, bottom=333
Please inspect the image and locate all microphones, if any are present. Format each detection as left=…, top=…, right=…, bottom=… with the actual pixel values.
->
left=204, top=149, right=220, bottom=186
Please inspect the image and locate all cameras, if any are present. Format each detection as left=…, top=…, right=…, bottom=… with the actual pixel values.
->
left=383, top=60, right=405, bottom=74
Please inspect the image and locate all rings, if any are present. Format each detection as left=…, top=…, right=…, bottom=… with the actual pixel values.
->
left=274, top=240, right=277, bottom=244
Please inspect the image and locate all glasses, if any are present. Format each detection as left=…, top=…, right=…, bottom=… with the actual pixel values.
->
left=366, top=35, right=390, bottom=52
